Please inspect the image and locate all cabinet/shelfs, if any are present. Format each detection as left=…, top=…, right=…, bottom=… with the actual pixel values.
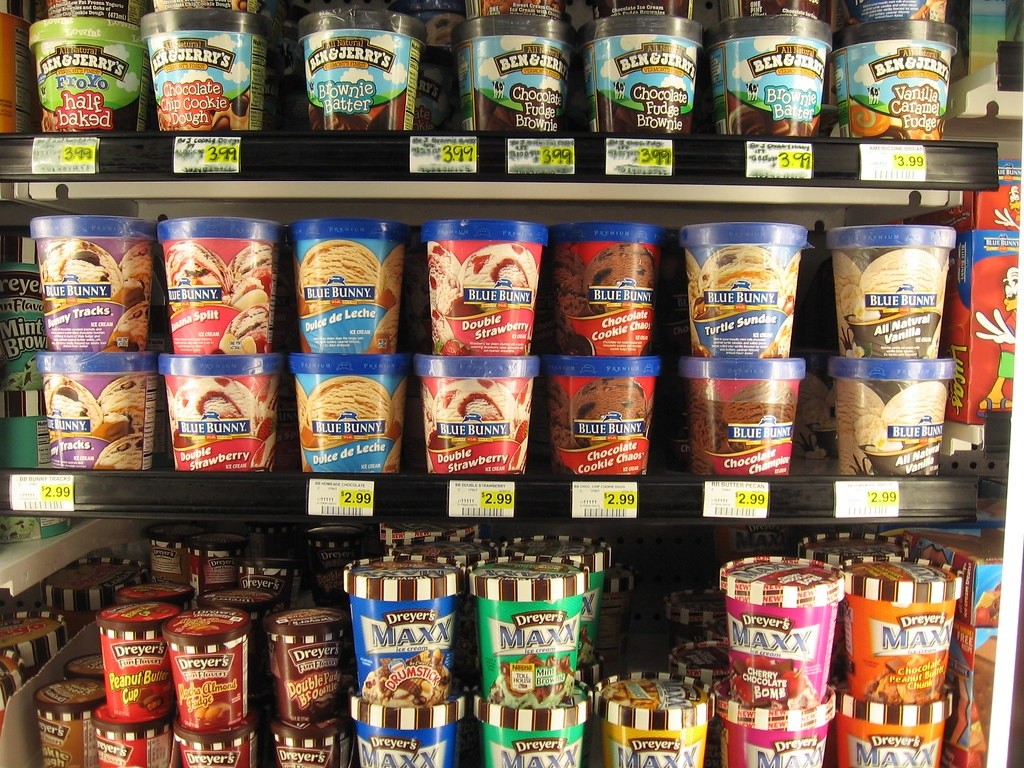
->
left=0, top=133, right=1023, bottom=768
